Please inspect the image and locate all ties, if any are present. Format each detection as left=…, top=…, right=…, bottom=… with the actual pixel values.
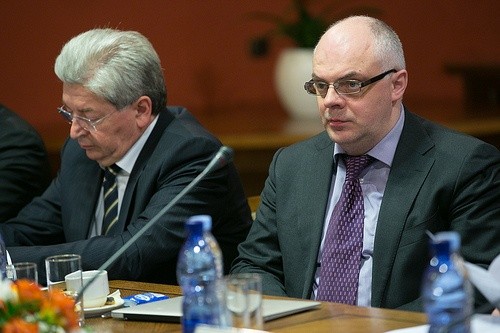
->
left=101, top=162, right=122, bottom=236
left=314, top=155, right=379, bottom=307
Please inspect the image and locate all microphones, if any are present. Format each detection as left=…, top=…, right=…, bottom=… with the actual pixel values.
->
left=76, top=146, right=235, bottom=303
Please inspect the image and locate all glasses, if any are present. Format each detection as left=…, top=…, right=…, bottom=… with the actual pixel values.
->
left=56, top=105, right=120, bottom=134
left=304, top=68, right=399, bottom=96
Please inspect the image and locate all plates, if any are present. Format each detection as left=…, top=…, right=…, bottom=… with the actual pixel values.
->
left=80, top=295, right=124, bottom=318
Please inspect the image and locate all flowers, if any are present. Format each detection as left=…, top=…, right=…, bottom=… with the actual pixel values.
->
left=0, top=278, right=84, bottom=333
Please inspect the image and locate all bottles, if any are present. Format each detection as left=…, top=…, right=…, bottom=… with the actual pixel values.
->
left=188, top=214, right=223, bottom=305
left=421, top=231, right=473, bottom=333
left=176, top=222, right=221, bottom=333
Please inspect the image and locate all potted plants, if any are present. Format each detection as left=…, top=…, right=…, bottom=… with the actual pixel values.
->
left=248, top=0, right=383, bottom=122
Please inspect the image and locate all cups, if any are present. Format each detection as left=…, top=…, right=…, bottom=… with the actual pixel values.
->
left=214, top=272, right=265, bottom=332
left=45, top=254, right=83, bottom=294
left=6, top=262, right=38, bottom=288
left=65, top=270, right=109, bottom=308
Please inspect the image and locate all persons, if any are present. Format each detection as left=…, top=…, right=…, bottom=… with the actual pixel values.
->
left=231, top=16, right=500, bottom=316
left=0, top=103, right=57, bottom=222
left=0, top=30, right=253, bottom=286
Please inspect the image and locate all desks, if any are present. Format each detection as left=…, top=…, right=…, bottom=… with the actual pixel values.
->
left=214, top=120, right=500, bottom=198
left=55, top=280, right=427, bottom=333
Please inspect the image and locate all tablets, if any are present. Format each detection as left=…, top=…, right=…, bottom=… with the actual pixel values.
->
left=111, top=295, right=321, bottom=325
